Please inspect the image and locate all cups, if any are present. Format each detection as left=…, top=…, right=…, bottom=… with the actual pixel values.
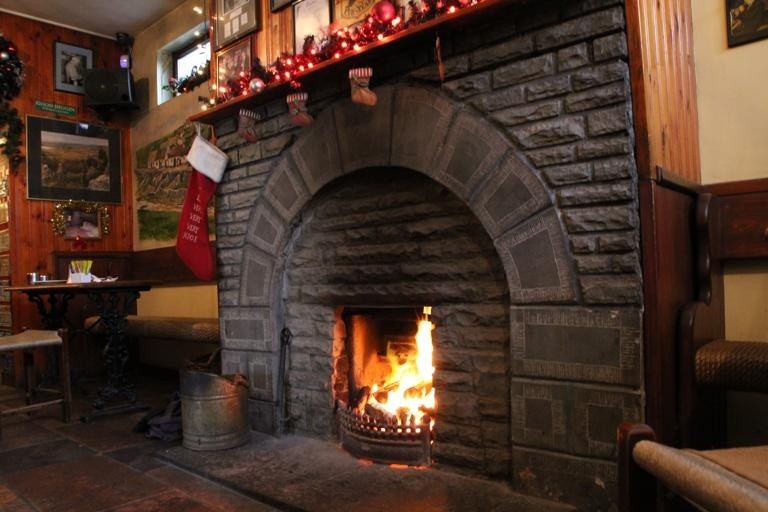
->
left=27, top=272, right=39, bottom=285
left=39, top=273, right=51, bottom=280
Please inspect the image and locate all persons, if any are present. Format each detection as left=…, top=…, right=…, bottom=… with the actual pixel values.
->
left=64, top=55, right=84, bottom=86
left=96, top=145, right=107, bottom=163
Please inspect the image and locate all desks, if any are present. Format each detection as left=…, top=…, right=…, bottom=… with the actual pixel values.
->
left=4, top=280, right=164, bottom=424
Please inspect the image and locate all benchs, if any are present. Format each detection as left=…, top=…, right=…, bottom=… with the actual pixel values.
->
left=673, top=183, right=768, bottom=450
left=121, top=279, right=222, bottom=390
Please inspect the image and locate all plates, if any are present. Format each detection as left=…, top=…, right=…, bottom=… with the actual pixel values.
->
left=33, top=280, right=67, bottom=285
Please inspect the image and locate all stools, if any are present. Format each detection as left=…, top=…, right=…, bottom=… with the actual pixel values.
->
left=615, top=420, right=768, bottom=512
left=0, top=326, right=73, bottom=424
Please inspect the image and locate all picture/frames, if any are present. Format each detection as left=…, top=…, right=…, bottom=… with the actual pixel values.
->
left=61, top=208, right=104, bottom=241
left=25, top=113, right=126, bottom=206
left=214, top=0, right=400, bottom=102
left=725, top=0, right=768, bottom=48
left=52, top=40, right=95, bottom=96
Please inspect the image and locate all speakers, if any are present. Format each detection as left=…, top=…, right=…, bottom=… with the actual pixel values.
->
left=83, top=66, right=136, bottom=105
